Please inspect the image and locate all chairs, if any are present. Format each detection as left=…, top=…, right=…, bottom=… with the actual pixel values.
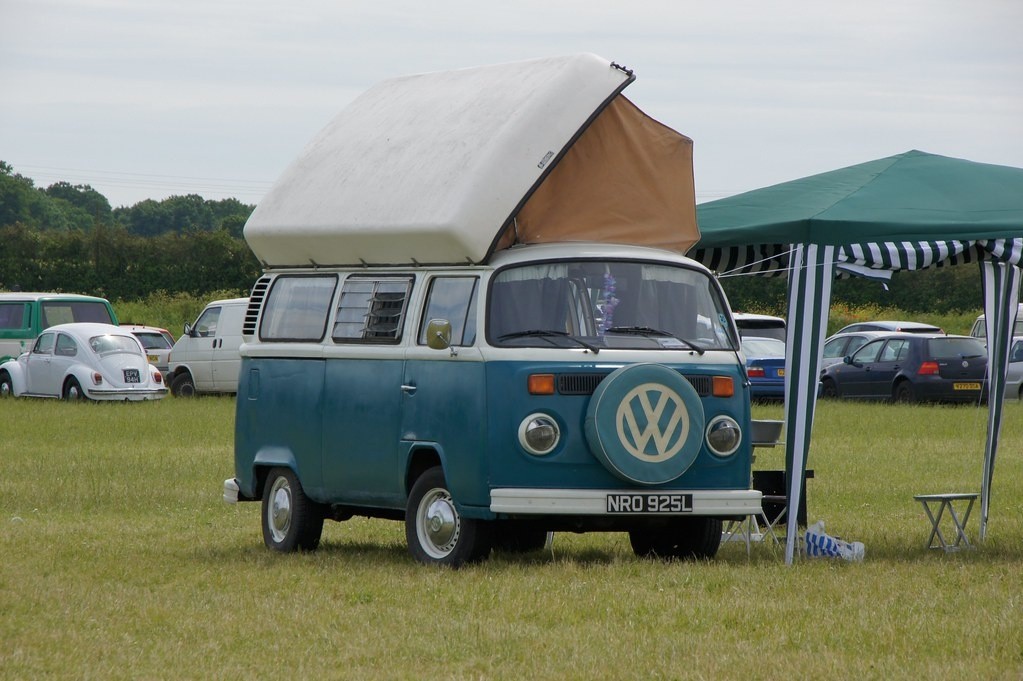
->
left=855, top=344, right=873, bottom=356
left=894, top=347, right=908, bottom=362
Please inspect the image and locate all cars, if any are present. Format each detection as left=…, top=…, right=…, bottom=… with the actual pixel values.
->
left=819, top=332, right=905, bottom=373
left=0, top=321, right=168, bottom=406
left=818, top=332, right=990, bottom=406
left=740, top=336, right=786, bottom=398
left=984, top=336, right=1023, bottom=401
left=834, top=321, right=944, bottom=337
left=121, top=326, right=176, bottom=347
left=120, top=327, right=175, bottom=383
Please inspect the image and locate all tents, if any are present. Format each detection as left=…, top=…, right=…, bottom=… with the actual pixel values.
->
left=696, top=149, right=1023, bottom=566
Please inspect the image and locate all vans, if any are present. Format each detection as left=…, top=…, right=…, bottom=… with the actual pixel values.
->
left=223, top=50, right=761, bottom=572
left=968, top=303, right=1023, bottom=338
left=166, top=297, right=250, bottom=401
left=0, top=292, right=121, bottom=361
left=718, top=312, right=786, bottom=348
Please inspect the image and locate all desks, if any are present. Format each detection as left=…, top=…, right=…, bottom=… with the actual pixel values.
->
left=913, top=493, right=982, bottom=553
left=719, top=494, right=804, bottom=566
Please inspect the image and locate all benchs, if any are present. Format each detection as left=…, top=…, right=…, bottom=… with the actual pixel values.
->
left=486, top=277, right=699, bottom=341
